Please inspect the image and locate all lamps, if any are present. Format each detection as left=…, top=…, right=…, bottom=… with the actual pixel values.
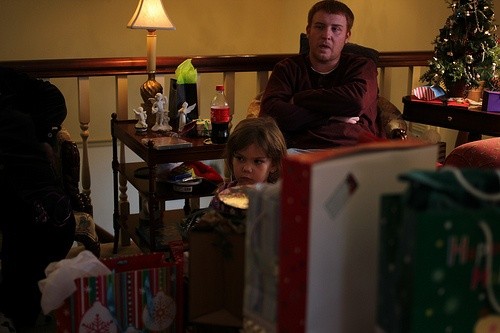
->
left=127, top=0, right=177, bottom=125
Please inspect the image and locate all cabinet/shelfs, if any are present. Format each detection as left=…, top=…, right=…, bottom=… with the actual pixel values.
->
left=107, top=112, right=232, bottom=255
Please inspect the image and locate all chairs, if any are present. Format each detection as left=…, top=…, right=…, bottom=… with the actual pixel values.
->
left=247, top=31, right=410, bottom=143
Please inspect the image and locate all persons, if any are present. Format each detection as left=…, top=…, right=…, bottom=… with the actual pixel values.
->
left=179, top=102, right=188, bottom=129
left=152, top=93, right=168, bottom=126
left=133, top=107, right=147, bottom=125
left=259, top=0, right=381, bottom=145
left=209, top=116, right=286, bottom=218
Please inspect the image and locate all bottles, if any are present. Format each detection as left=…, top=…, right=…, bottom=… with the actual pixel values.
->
left=211, top=85, right=230, bottom=144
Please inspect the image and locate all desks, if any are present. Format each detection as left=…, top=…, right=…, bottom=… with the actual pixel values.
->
left=401, top=94, right=500, bottom=148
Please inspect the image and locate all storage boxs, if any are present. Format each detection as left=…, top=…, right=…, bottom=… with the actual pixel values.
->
left=51, top=255, right=187, bottom=333
